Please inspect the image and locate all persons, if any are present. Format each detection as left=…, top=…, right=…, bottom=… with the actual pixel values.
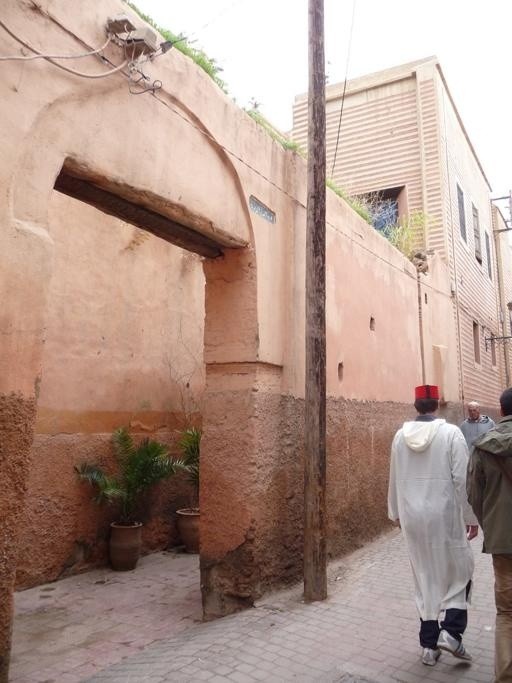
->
left=387, top=384, right=480, bottom=666
left=459, top=401, right=495, bottom=453
left=466, top=387, right=511, bottom=682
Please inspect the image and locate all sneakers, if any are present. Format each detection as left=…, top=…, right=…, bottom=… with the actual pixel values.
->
left=422, top=629, right=472, bottom=665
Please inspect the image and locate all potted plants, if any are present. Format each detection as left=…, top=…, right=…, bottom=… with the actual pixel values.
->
left=168, top=425, right=201, bottom=554
left=68, top=424, right=190, bottom=571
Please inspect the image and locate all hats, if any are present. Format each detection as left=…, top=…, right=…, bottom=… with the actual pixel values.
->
left=415, top=385, right=439, bottom=400
